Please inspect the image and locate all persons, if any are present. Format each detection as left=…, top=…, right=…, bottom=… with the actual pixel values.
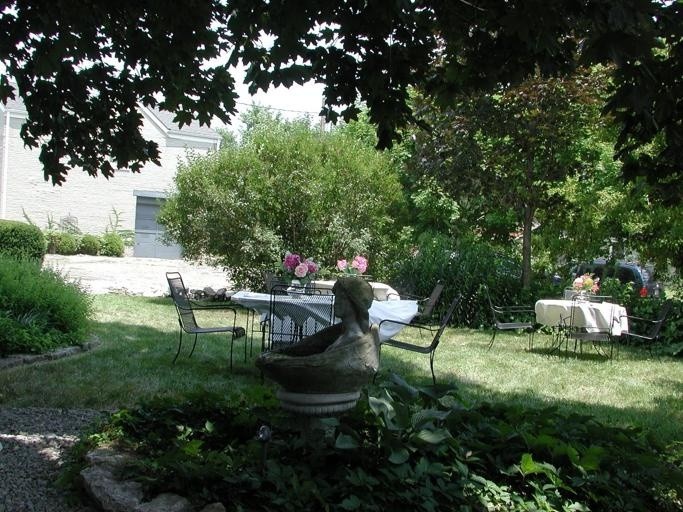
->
left=272, top=275, right=373, bottom=363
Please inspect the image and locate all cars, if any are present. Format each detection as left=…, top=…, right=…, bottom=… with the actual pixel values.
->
left=549, top=258, right=666, bottom=302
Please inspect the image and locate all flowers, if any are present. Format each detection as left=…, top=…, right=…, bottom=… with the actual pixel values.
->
left=280, top=250, right=318, bottom=285
left=572, top=272, right=601, bottom=295
left=336, top=255, right=368, bottom=278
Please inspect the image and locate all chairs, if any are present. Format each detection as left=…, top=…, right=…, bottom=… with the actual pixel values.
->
left=164, top=271, right=462, bottom=387
left=482, top=284, right=671, bottom=367
